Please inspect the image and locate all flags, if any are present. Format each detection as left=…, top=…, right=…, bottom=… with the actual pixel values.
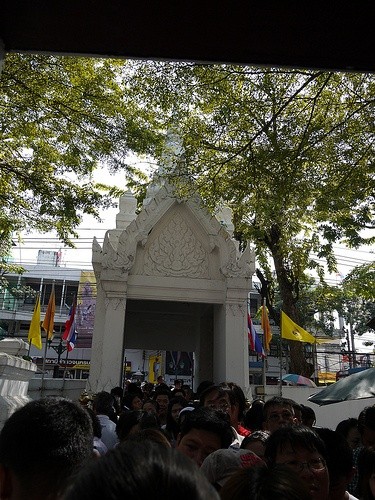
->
left=28, top=284, right=78, bottom=353
left=248, top=306, right=319, bottom=359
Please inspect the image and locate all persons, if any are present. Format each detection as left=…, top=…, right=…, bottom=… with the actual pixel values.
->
left=0, top=375, right=375, bottom=500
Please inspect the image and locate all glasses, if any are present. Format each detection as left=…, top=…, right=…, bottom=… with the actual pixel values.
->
left=275, top=456, right=327, bottom=473
left=269, top=413, right=293, bottom=422
left=208, top=403, right=229, bottom=409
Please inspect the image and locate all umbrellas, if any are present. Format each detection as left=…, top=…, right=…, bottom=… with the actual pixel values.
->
left=277, top=367, right=375, bottom=408
